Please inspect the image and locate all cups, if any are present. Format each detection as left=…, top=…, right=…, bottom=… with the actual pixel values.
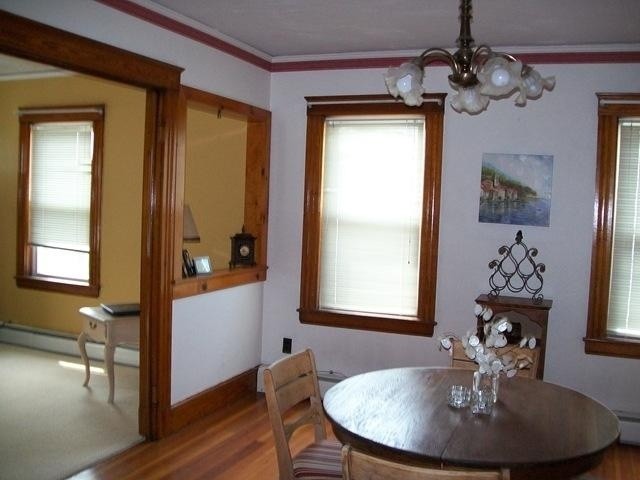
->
left=471, top=371, right=494, bottom=416
left=447, top=384, right=471, bottom=408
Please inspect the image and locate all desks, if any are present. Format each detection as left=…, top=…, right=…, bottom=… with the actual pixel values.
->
left=323, top=368, right=621, bottom=480
left=475, top=293, right=553, bottom=380
left=77, top=308, right=140, bottom=404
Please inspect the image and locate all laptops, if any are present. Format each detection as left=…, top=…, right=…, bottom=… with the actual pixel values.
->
left=101, top=303, right=140, bottom=316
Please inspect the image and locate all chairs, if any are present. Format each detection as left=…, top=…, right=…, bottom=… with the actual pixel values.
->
left=341, top=444, right=511, bottom=479
left=263, top=349, right=343, bottom=480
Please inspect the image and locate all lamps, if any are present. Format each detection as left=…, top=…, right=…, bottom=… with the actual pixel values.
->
left=383, top=0, right=557, bottom=115
left=183, top=204, right=200, bottom=242
